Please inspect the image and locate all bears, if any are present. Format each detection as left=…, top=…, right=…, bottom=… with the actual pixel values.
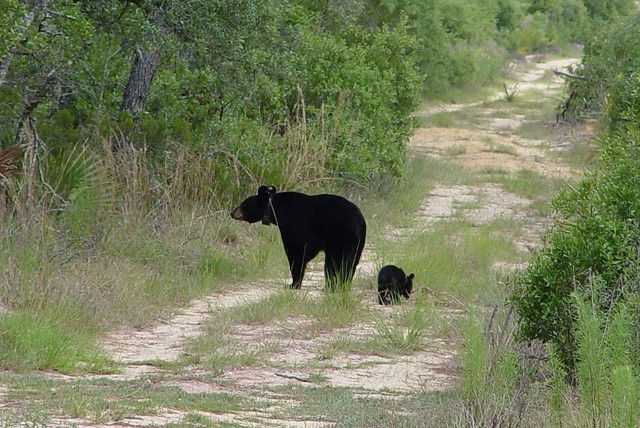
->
left=230, top=184, right=366, bottom=292
left=378, top=264, right=414, bottom=304
